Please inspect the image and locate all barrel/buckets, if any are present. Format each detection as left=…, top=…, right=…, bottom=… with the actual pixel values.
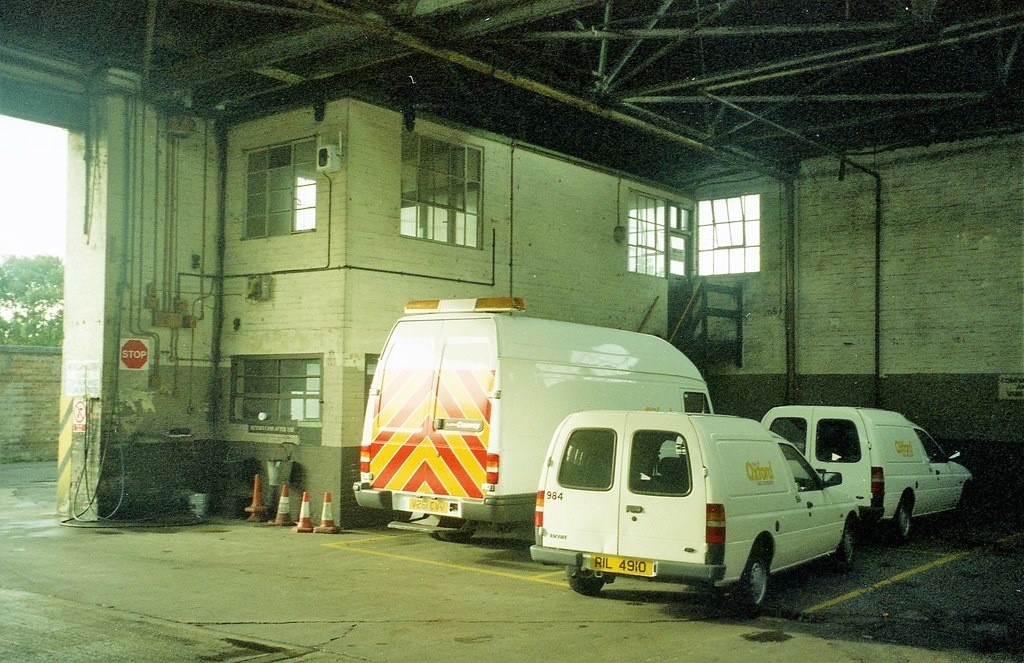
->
left=187, top=493, right=207, bottom=515
left=267, top=459, right=292, bottom=486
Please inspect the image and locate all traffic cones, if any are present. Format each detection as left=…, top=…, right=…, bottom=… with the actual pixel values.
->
left=296, top=491, right=315, bottom=533
left=244, top=474, right=269, bottom=523
left=269, top=484, right=297, bottom=527
left=313, top=490, right=341, bottom=534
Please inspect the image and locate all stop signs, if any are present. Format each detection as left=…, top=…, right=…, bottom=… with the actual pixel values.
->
left=121, top=339, right=149, bottom=369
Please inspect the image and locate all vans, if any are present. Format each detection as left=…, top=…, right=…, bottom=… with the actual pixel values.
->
left=529, top=410, right=863, bottom=618
left=351, top=296, right=715, bottom=543
left=758, top=405, right=984, bottom=545
